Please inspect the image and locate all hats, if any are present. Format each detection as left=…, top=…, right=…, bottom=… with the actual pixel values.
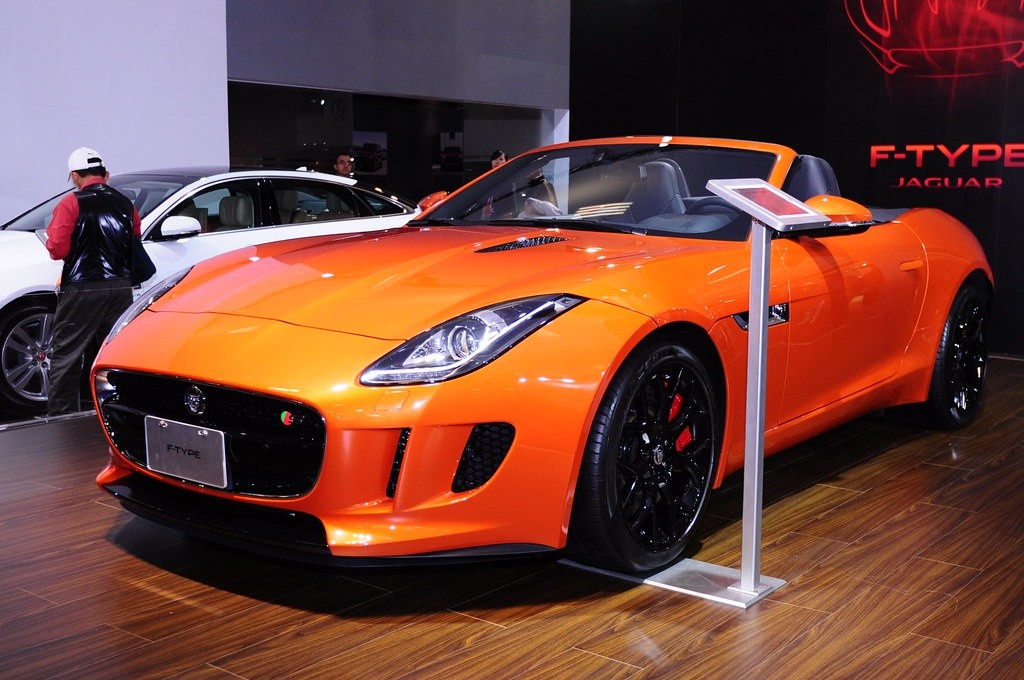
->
left=65, top=147, right=104, bottom=183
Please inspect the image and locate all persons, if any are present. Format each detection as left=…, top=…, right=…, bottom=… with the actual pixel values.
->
left=32, top=147, right=142, bottom=420
left=526, top=167, right=559, bottom=208
left=481, top=149, right=518, bottom=219
left=333, top=150, right=352, bottom=178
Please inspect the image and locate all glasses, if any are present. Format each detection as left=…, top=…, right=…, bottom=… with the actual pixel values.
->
left=337, top=160, right=352, bottom=166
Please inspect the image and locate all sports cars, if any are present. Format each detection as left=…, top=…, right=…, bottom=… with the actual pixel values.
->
left=0, top=165, right=429, bottom=420
left=80, top=133, right=1004, bottom=578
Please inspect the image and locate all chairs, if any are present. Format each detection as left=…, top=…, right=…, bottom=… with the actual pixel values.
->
left=601, top=159, right=693, bottom=225
left=780, top=155, right=841, bottom=205
left=168, top=198, right=199, bottom=221
left=215, top=194, right=256, bottom=232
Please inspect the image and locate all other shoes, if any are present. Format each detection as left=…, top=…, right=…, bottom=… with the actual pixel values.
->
left=36, top=413, right=56, bottom=419
